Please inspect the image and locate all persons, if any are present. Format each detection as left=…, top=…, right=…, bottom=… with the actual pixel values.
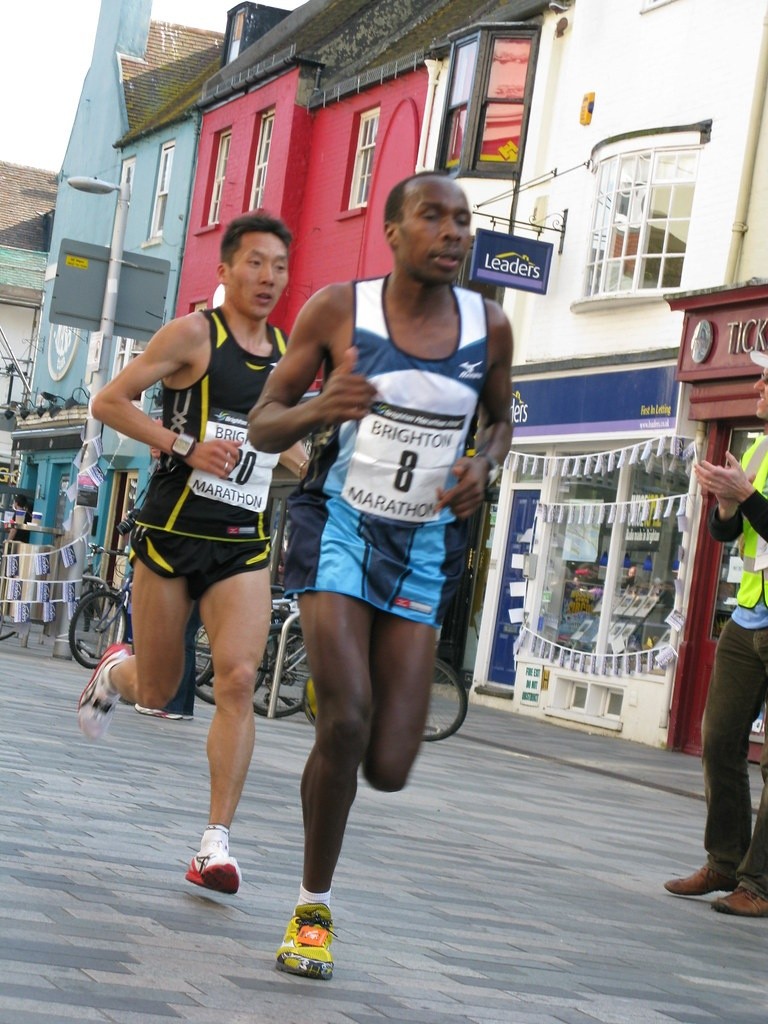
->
left=6, top=494, right=32, bottom=544
left=665, top=348, right=768, bottom=916
left=245, top=173, right=515, bottom=981
left=79, top=212, right=311, bottom=895
left=134, top=415, right=201, bottom=720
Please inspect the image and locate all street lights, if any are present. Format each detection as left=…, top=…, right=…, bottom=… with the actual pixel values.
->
left=52, top=176, right=131, bottom=661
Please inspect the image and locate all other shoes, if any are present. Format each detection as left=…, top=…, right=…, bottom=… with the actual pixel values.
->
left=183, top=714, right=193, bottom=720
left=136, top=703, right=182, bottom=719
left=711, top=886, right=768, bottom=917
left=663, top=866, right=739, bottom=896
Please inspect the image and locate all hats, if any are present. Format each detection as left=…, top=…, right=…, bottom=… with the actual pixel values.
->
left=15, top=493, right=26, bottom=506
left=750, top=351, right=768, bottom=368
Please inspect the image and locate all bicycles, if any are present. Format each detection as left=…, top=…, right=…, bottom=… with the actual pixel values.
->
left=68, top=541, right=468, bottom=741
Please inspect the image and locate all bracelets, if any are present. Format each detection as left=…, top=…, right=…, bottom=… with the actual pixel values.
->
left=298, top=460, right=309, bottom=478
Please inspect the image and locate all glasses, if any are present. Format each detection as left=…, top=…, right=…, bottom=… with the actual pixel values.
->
left=761, top=372, right=768, bottom=384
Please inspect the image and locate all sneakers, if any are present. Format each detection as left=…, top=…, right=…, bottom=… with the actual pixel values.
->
left=276, top=904, right=338, bottom=980
left=79, top=645, right=131, bottom=740
left=302, top=676, right=319, bottom=726
left=185, top=841, right=241, bottom=894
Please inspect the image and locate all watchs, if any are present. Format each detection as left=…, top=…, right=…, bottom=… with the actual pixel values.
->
left=171, top=434, right=197, bottom=461
left=475, top=452, right=501, bottom=486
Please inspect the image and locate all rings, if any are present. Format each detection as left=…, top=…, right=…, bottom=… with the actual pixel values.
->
left=708, top=481, right=712, bottom=486
left=223, top=462, right=228, bottom=471
left=225, top=451, right=230, bottom=461
